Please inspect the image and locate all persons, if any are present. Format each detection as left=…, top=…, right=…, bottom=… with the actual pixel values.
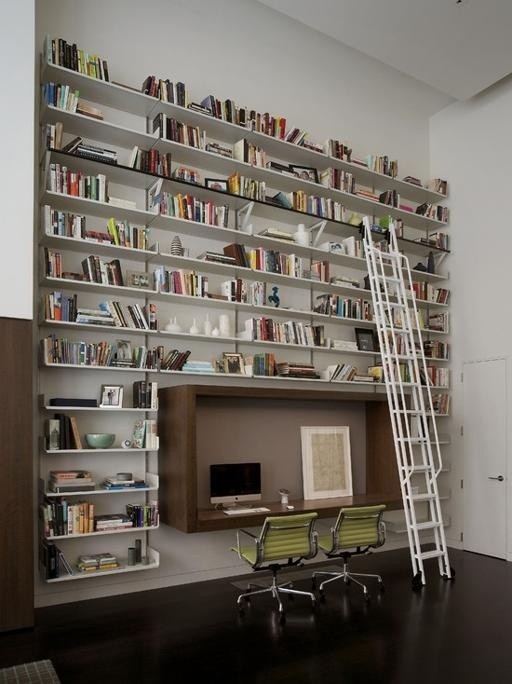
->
left=108, top=390, right=116, bottom=405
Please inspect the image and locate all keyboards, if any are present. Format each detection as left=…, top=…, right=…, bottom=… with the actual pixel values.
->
left=223, top=507, right=271, bottom=515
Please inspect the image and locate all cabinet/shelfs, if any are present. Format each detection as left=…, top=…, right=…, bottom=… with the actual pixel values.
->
left=31, top=33, right=453, bottom=608
left=158, top=383, right=412, bottom=534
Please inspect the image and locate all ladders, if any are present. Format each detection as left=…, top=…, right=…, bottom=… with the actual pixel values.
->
left=359, top=216, right=456, bottom=592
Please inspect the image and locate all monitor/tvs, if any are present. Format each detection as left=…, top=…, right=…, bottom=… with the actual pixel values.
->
left=209, top=463, right=261, bottom=510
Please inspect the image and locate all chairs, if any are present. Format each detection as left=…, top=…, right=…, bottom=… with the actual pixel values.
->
left=317, top=504, right=387, bottom=601
left=227, top=512, right=320, bottom=615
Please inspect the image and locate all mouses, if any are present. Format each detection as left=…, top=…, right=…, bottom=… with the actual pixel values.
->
left=286, top=505, right=294, bottom=509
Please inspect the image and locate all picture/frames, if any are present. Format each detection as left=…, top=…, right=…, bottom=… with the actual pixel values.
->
left=99, top=383, right=124, bottom=408
left=300, top=425, right=354, bottom=501
left=288, top=164, right=318, bottom=183
left=205, top=178, right=229, bottom=192
left=354, top=327, right=378, bottom=352
left=223, top=352, right=246, bottom=374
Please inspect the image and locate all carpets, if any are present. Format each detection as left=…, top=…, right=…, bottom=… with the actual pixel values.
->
left=0, top=659, right=60, bottom=683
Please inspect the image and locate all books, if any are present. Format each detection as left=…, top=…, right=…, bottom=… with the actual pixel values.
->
left=54, top=414, right=83, bottom=449
left=39, top=35, right=453, bottom=387
left=76, top=553, right=117, bottom=572
left=133, top=381, right=154, bottom=408
left=43, top=500, right=158, bottom=537
left=144, top=419, right=156, bottom=448
left=44, top=541, right=73, bottom=579
left=426, top=394, right=450, bottom=414
left=100, top=477, right=149, bottom=490
left=48, top=471, right=95, bottom=493
